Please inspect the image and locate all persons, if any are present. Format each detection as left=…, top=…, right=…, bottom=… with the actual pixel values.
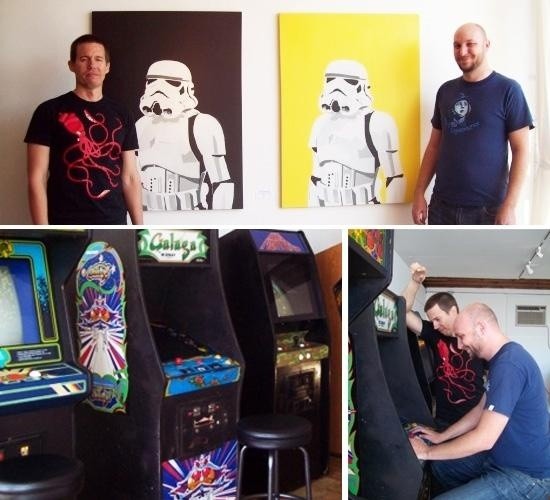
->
left=401, top=262, right=490, bottom=434
left=405, top=301, right=550, bottom=500
left=22, top=34, right=145, bottom=225
left=412, top=23, right=537, bottom=226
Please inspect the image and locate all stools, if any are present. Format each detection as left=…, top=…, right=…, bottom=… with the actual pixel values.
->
left=234, top=414, right=317, bottom=498
left=0, top=455, right=88, bottom=499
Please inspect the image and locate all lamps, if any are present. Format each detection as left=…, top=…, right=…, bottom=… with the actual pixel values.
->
left=525, top=244, right=544, bottom=275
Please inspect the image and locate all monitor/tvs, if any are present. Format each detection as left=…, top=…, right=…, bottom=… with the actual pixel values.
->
left=0, top=255, right=41, bottom=347
left=151, top=325, right=205, bottom=363
left=269, top=265, right=324, bottom=318
